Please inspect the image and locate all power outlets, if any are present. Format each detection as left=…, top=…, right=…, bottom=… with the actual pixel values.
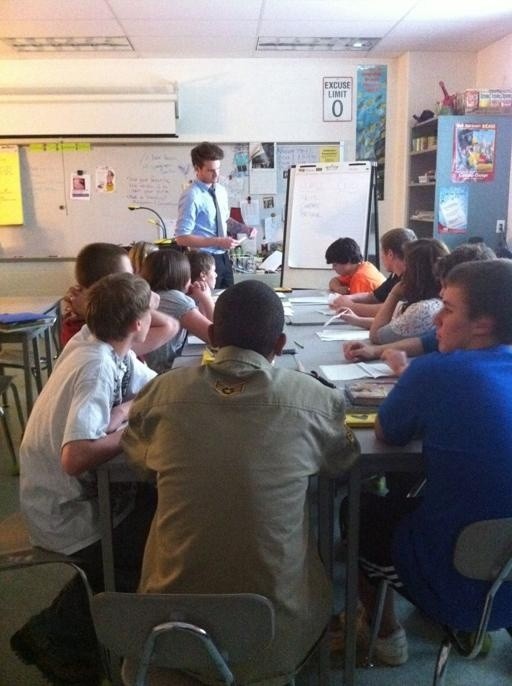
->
left=496, top=220, right=505, bottom=234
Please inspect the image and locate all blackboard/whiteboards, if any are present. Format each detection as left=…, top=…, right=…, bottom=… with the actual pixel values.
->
left=279, top=161, right=376, bottom=291
left=0, top=138, right=344, bottom=262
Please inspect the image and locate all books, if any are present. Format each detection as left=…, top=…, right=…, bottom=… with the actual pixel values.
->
left=411, top=135, right=437, bottom=152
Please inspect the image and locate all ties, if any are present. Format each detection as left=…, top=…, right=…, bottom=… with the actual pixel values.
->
left=209, top=188, right=224, bottom=236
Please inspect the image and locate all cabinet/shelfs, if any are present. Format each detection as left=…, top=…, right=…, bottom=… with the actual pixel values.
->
left=404, top=114, right=512, bottom=250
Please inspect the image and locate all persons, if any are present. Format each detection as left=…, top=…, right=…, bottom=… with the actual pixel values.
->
left=11, top=226, right=512, bottom=686
left=233, top=147, right=248, bottom=174
left=457, top=129, right=475, bottom=173
left=245, top=196, right=255, bottom=223
left=104, top=171, right=114, bottom=190
left=174, top=142, right=257, bottom=290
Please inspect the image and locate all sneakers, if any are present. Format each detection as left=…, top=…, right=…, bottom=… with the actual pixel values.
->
left=339, top=599, right=408, bottom=665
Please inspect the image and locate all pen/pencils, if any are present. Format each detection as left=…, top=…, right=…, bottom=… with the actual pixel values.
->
left=238, top=236, right=248, bottom=243
left=294, top=340, right=304, bottom=348
left=324, top=311, right=347, bottom=327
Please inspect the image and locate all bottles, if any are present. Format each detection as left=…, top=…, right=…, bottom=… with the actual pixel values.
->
left=229, top=246, right=255, bottom=273
left=411, top=135, right=436, bottom=153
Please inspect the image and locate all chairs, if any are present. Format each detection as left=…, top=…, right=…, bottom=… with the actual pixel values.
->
left=0, top=549, right=111, bottom=686
left=91, top=590, right=275, bottom=686
left=365, top=518, right=512, bottom=686
left=0, top=376, right=26, bottom=476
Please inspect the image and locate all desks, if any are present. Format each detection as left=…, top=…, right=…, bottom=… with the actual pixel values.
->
left=0, top=296, right=64, bottom=417
left=96, top=289, right=423, bottom=686
